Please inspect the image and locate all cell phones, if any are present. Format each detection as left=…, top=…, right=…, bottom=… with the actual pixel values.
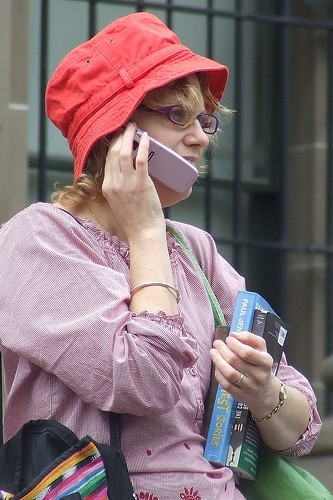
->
left=103, top=123, right=199, bottom=194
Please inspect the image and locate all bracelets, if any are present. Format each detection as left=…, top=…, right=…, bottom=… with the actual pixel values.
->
left=129, top=282, right=181, bottom=304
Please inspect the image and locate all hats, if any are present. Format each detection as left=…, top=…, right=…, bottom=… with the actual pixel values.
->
left=45, top=11, right=229, bottom=185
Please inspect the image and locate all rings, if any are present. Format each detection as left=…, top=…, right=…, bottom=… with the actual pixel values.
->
left=235, top=372, right=245, bottom=384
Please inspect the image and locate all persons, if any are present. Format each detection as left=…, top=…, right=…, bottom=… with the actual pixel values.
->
left=0, top=12, right=322, bottom=500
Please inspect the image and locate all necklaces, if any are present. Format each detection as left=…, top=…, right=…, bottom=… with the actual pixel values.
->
left=83, top=198, right=113, bottom=238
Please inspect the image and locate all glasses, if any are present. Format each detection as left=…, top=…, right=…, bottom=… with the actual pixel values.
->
left=137, top=102, right=219, bottom=135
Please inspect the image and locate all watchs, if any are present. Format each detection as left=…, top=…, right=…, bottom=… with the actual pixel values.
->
left=252, top=381, right=288, bottom=422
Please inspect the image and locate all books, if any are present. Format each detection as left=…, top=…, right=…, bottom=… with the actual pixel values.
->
left=202, top=289, right=292, bottom=483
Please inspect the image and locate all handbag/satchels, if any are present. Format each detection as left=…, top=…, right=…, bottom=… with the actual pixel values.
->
left=165, top=223, right=333, bottom=499
left=0, top=205, right=138, bottom=500
